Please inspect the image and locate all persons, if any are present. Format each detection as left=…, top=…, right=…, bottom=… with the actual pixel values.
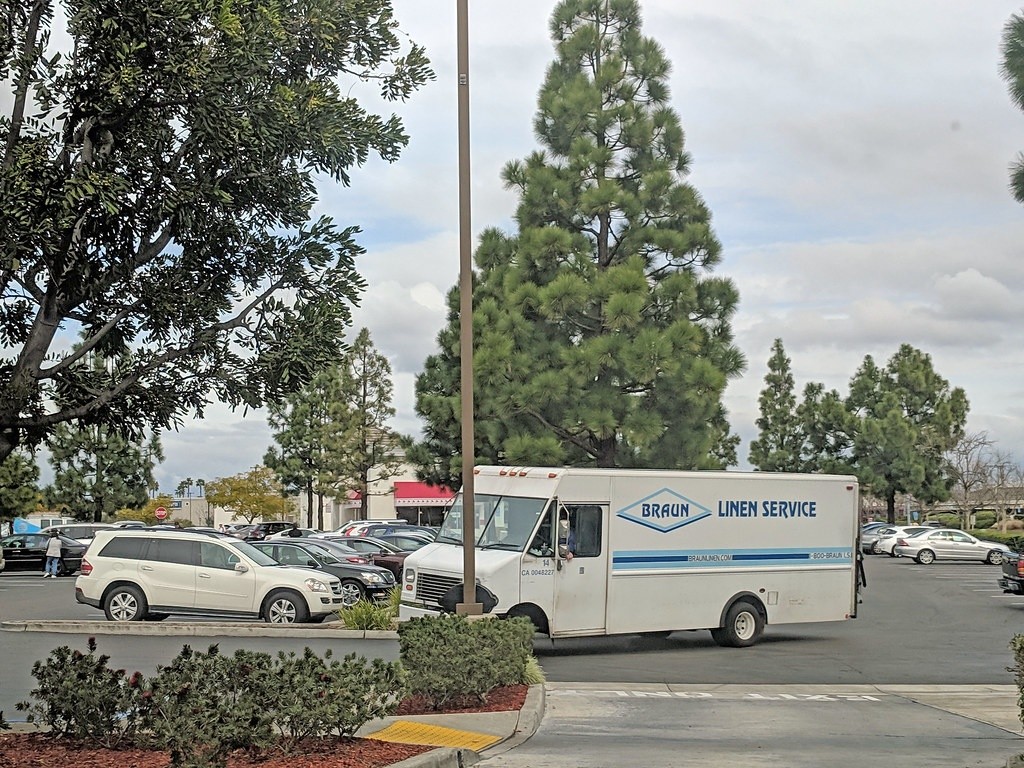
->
left=44, top=532, right=62, bottom=578
left=175, top=521, right=180, bottom=529
left=286, top=549, right=306, bottom=564
left=1, top=520, right=10, bottom=537
left=550, top=526, right=575, bottom=559
left=288, top=523, right=302, bottom=537
left=218, top=523, right=226, bottom=533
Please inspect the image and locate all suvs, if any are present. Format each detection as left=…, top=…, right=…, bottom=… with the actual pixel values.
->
left=0, top=512, right=439, bottom=633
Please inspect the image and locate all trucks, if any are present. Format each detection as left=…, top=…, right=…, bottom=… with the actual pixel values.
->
left=395, top=464, right=863, bottom=650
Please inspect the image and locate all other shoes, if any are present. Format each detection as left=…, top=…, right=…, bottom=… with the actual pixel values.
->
left=44, top=572, right=50, bottom=578
left=50, top=575, right=58, bottom=579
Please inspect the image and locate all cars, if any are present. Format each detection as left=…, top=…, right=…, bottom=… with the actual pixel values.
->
left=860, top=522, right=936, bottom=559
left=898, top=528, right=1011, bottom=565
